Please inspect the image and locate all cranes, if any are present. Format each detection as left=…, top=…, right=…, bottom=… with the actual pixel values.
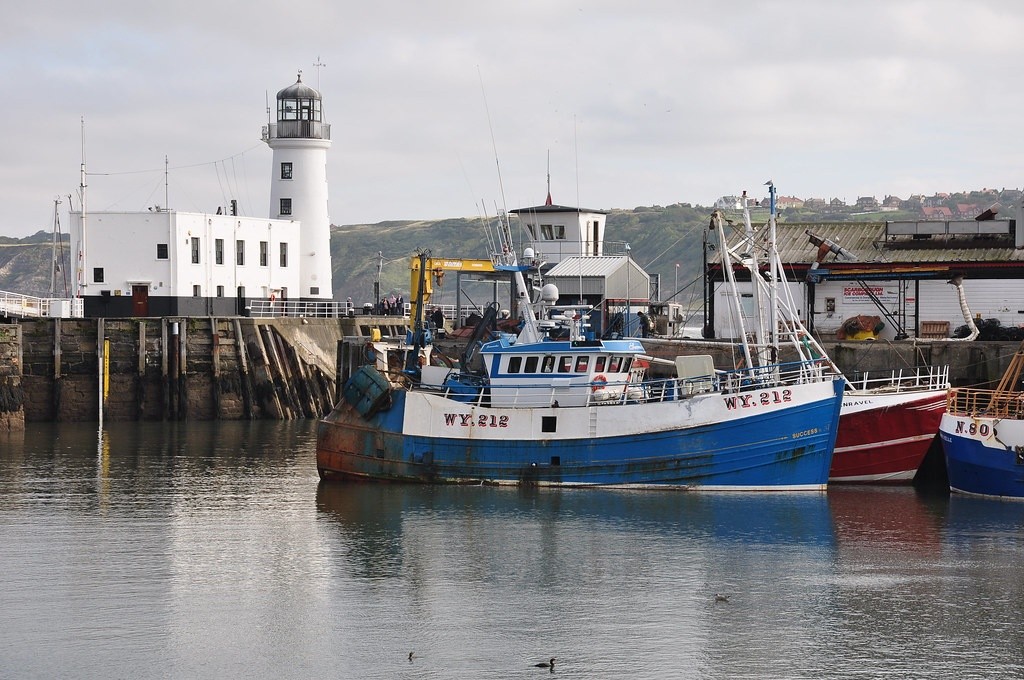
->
left=408, top=246, right=502, bottom=344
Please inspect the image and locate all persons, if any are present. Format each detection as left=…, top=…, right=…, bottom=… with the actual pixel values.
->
left=380, top=293, right=403, bottom=317
left=347, top=297, right=351, bottom=315
left=426, top=306, right=443, bottom=329
left=637, top=311, right=649, bottom=338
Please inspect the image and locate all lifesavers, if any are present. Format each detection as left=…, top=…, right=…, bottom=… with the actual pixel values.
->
left=593, top=375, right=607, bottom=391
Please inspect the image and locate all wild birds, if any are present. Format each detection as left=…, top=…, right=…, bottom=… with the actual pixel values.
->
left=714, top=593, right=731, bottom=601
left=534, top=659, right=556, bottom=667
left=408, top=651, right=424, bottom=661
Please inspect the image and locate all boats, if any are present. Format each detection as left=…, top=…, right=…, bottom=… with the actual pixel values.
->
left=939, top=337, right=1024, bottom=503
left=316, top=181, right=846, bottom=496
left=622, top=182, right=960, bottom=481
